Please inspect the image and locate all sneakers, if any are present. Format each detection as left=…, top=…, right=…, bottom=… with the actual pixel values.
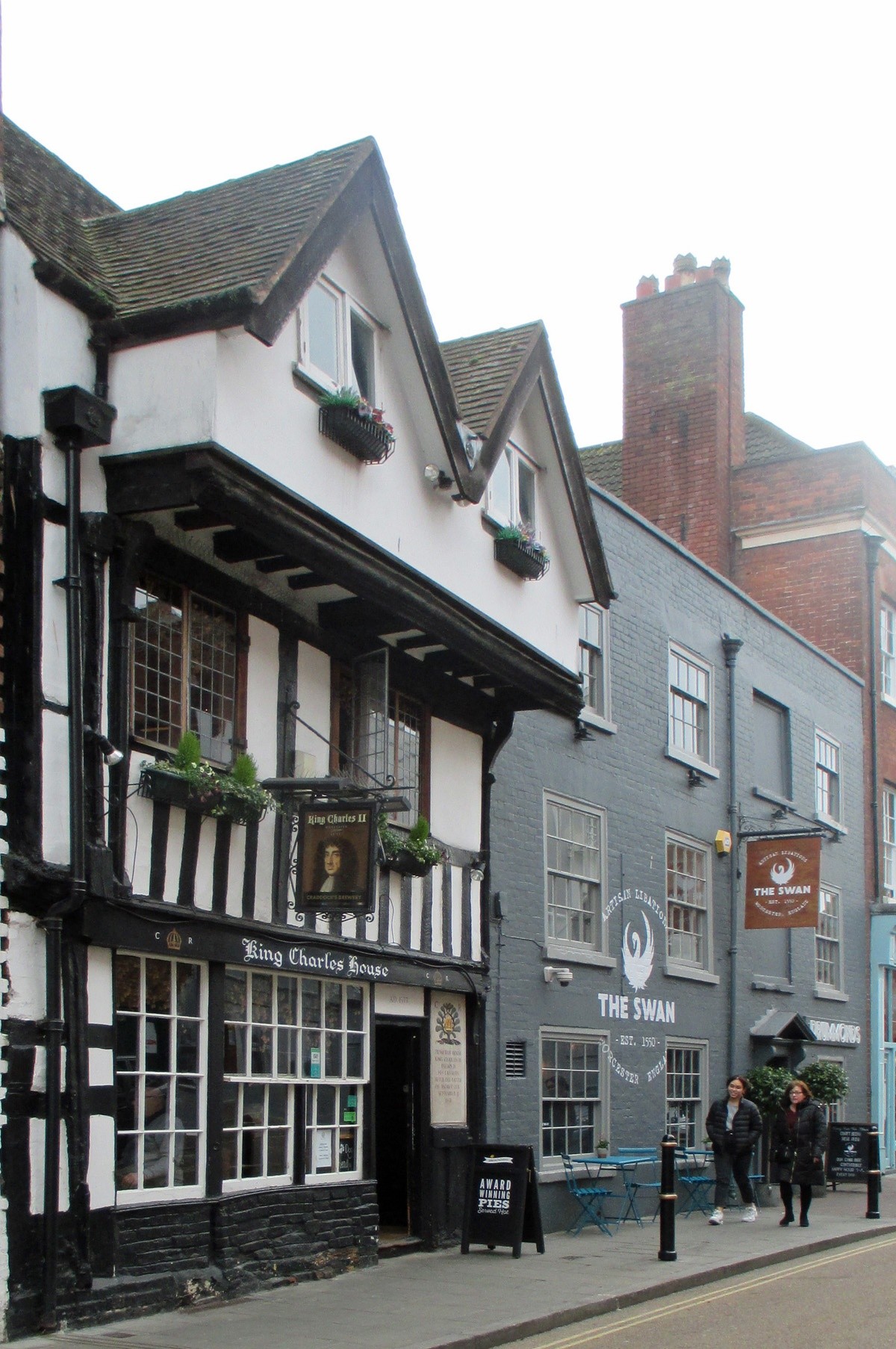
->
left=743, top=1206, right=759, bottom=1222
left=709, top=1208, right=727, bottom=1227
left=779, top=1215, right=795, bottom=1225
left=799, top=1214, right=810, bottom=1226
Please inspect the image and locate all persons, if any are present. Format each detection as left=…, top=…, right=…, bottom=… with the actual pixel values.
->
left=773, top=1081, right=827, bottom=1226
left=113, top=1087, right=184, bottom=1190
left=706, top=1075, right=762, bottom=1224
left=542, top=1076, right=575, bottom=1156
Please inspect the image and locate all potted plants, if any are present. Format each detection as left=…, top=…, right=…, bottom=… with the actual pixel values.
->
left=794, top=1060, right=852, bottom=1197
left=495, top=517, right=549, bottom=579
left=318, top=383, right=395, bottom=463
left=138, top=727, right=272, bottom=822
left=700, top=1137, right=714, bottom=1151
left=594, top=1139, right=609, bottom=1158
left=743, top=1063, right=798, bottom=1205
left=376, top=810, right=441, bottom=879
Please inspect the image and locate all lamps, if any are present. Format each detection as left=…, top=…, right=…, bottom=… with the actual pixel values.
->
left=574, top=718, right=597, bottom=741
left=469, top=858, right=487, bottom=881
left=771, top=810, right=788, bottom=820
left=827, top=830, right=843, bottom=844
left=688, top=769, right=706, bottom=787
left=83, top=722, right=125, bottom=766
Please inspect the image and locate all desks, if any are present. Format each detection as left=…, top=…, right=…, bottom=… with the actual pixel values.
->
left=684, top=1149, right=741, bottom=1213
left=571, top=1154, right=651, bottom=1234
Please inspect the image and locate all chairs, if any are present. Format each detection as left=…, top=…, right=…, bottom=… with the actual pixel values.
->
left=559, top=1152, right=614, bottom=1239
left=733, top=1173, right=765, bottom=1212
left=672, top=1145, right=718, bottom=1219
left=618, top=1146, right=662, bottom=1229
